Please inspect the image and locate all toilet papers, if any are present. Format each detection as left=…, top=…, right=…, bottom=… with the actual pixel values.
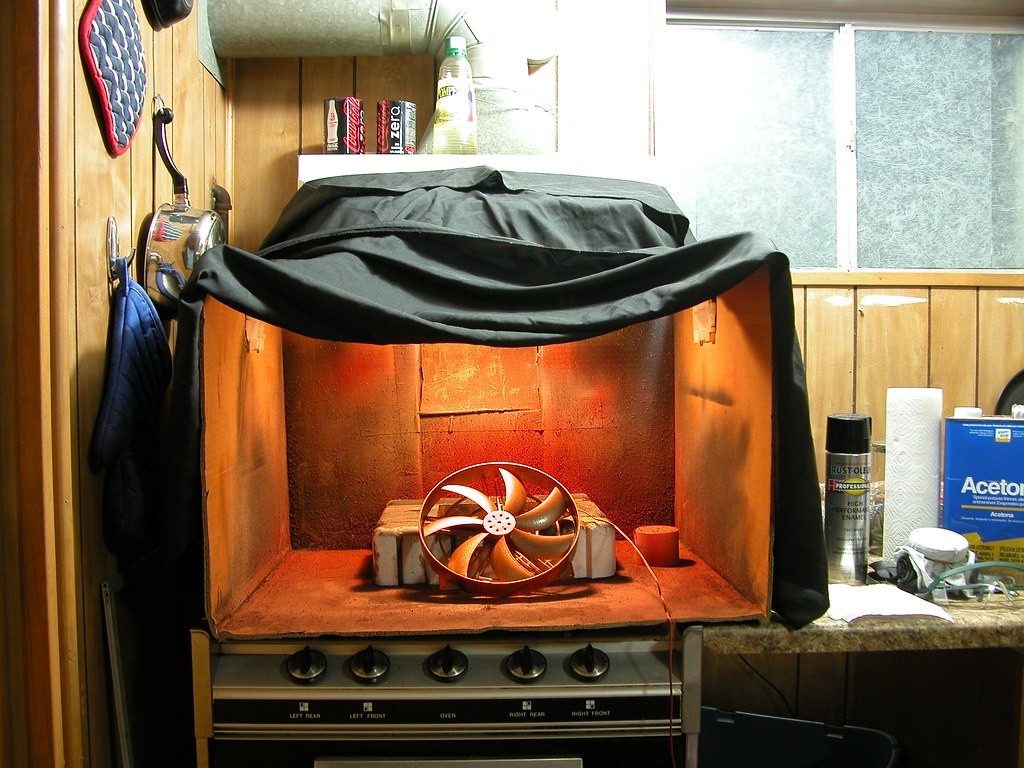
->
left=883, top=387, right=943, bottom=566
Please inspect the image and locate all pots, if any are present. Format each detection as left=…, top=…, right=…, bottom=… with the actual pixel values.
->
left=140, top=107, right=225, bottom=322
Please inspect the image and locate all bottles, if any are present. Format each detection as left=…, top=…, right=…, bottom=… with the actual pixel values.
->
left=432, top=35, right=478, bottom=155
left=821, top=412, right=873, bottom=587
